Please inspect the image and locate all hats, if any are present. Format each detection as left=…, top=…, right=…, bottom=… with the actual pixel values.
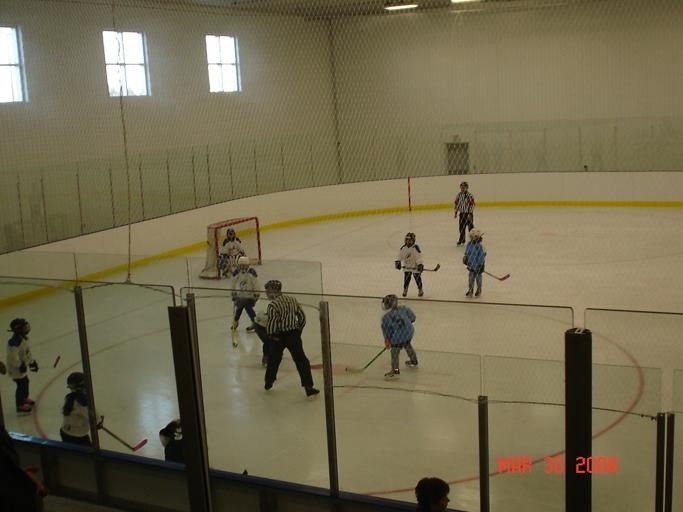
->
left=460, top=181, right=468, bottom=192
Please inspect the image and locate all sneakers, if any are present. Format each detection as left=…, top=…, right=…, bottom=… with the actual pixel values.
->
left=383, top=369, right=399, bottom=377
left=401, top=289, right=408, bottom=296
left=456, top=238, right=465, bottom=245
left=466, top=288, right=481, bottom=297
left=404, top=359, right=418, bottom=366
left=263, top=381, right=274, bottom=390
left=15, top=396, right=36, bottom=414
left=416, top=288, right=424, bottom=296
left=304, top=384, right=321, bottom=397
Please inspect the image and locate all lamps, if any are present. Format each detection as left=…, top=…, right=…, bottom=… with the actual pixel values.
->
left=384, top=0, right=420, bottom=11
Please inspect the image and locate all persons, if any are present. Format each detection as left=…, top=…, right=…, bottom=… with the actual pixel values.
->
left=464, top=230, right=487, bottom=297
left=6, top=317, right=39, bottom=412
left=381, top=294, right=418, bottom=378
left=60, top=371, right=103, bottom=447
left=264, top=279, right=320, bottom=397
left=395, top=232, right=424, bottom=297
left=218, top=228, right=247, bottom=279
left=454, top=182, right=482, bottom=245
left=254, top=311, right=271, bottom=368
left=415, top=477, right=450, bottom=512
left=160, top=420, right=184, bottom=465
left=232, top=256, right=261, bottom=331
left=0, top=395, right=48, bottom=512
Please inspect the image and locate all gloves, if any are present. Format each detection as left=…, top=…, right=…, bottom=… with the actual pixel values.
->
left=416, top=264, right=424, bottom=272
left=382, top=338, right=393, bottom=350
left=17, top=360, right=39, bottom=373
left=394, top=260, right=402, bottom=270
left=462, top=255, right=467, bottom=266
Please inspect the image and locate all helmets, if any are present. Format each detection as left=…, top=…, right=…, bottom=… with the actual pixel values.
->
left=66, top=372, right=86, bottom=390
left=264, top=279, right=282, bottom=301
left=225, top=227, right=236, bottom=240
left=5, top=317, right=31, bottom=334
left=237, top=256, right=249, bottom=266
left=404, top=232, right=415, bottom=240
left=468, top=227, right=483, bottom=239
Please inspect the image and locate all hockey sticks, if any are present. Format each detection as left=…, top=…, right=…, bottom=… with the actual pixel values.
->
left=309, top=363, right=324, bottom=373
left=401, top=264, right=440, bottom=271
left=484, top=271, right=510, bottom=281
left=232, top=301, right=238, bottom=347
left=101, top=427, right=148, bottom=451
left=346, top=346, right=386, bottom=373
left=37, top=356, right=60, bottom=369
